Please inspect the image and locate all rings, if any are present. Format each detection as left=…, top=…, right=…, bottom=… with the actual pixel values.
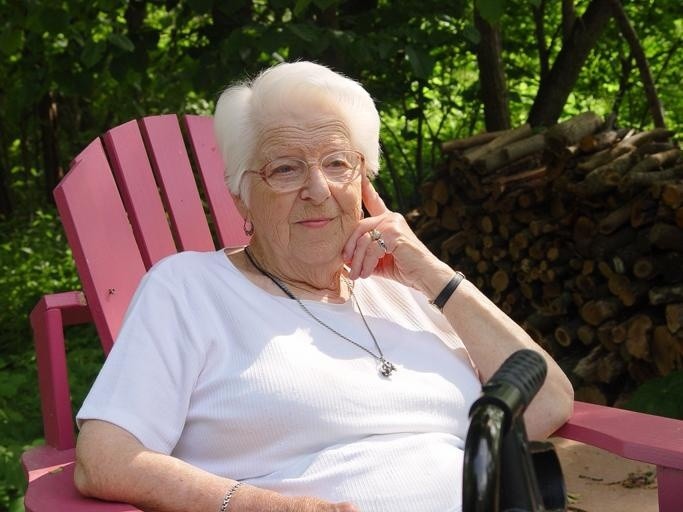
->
left=377, top=239, right=386, bottom=254
left=369, top=229, right=381, bottom=240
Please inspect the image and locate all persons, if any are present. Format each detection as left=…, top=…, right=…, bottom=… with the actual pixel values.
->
left=72, top=60, right=575, bottom=512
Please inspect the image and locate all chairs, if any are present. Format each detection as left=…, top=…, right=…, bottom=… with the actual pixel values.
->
left=20, top=114, right=683, bottom=512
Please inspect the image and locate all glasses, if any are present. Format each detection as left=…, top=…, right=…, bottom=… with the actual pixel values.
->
left=244, top=150, right=365, bottom=193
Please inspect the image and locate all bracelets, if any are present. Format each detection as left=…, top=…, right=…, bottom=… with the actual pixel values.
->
left=429, top=270, right=465, bottom=313
left=220, top=482, right=244, bottom=512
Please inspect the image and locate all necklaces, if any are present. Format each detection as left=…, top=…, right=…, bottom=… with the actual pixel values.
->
left=245, top=245, right=397, bottom=377
